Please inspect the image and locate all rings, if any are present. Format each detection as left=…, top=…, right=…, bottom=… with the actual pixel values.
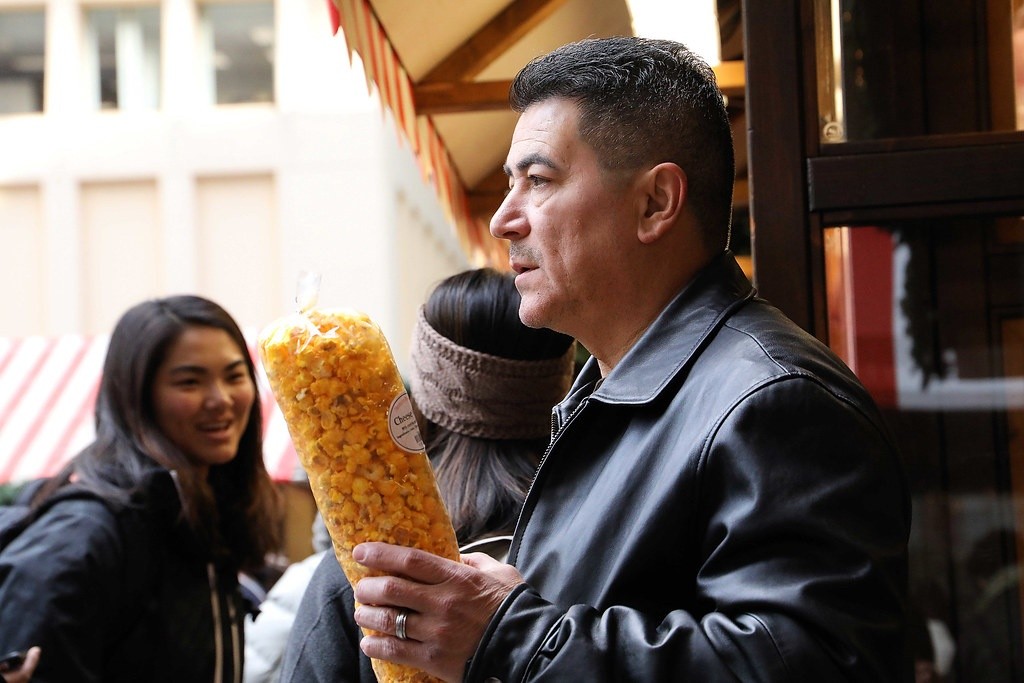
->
left=397, top=611, right=409, bottom=640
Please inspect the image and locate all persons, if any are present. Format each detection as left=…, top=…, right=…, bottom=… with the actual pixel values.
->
left=945, top=525, right=1023, bottom=683
left=275, top=265, right=577, bottom=682
left=349, top=34, right=919, bottom=683
left=1, top=293, right=292, bottom=682
left=229, top=482, right=338, bottom=682
